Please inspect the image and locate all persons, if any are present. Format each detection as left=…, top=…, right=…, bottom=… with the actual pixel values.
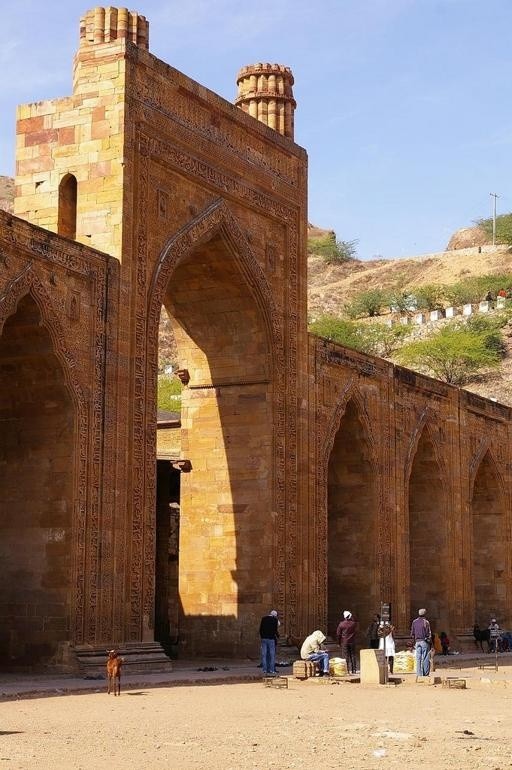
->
left=300, top=629, right=333, bottom=678
left=499, top=288, right=506, bottom=297
left=377, top=618, right=396, bottom=674
left=440, top=631, right=450, bottom=656
left=488, top=619, right=505, bottom=653
left=473, top=624, right=484, bottom=653
left=366, top=613, right=379, bottom=648
left=486, top=292, right=494, bottom=310
left=336, top=610, right=359, bottom=676
left=259, top=610, right=280, bottom=674
left=409, top=608, right=432, bottom=676
left=478, top=247, right=481, bottom=253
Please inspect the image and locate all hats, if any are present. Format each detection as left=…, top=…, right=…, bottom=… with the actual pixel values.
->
left=343, top=610, right=352, bottom=620
left=418, top=608, right=427, bottom=616
left=270, top=610, right=278, bottom=617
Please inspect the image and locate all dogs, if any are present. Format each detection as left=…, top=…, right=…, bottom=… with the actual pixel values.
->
left=106, top=649, right=123, bottom=696
